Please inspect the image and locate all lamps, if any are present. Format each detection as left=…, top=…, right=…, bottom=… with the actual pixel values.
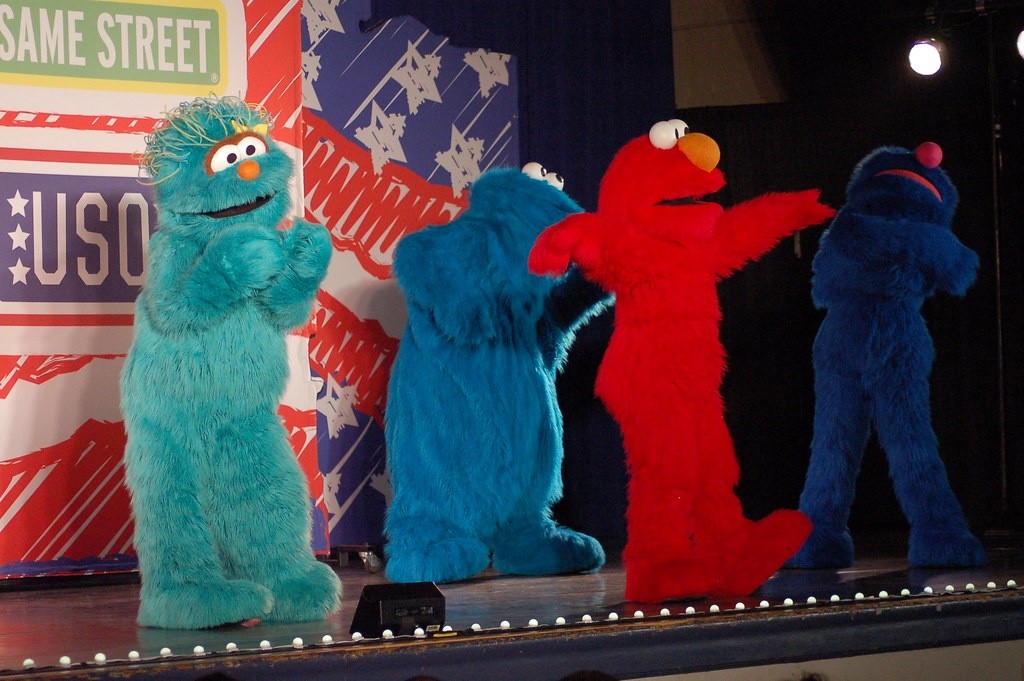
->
left=348, top=582, right=446, bottom=639
left=908, top=39, right=942, bottom=77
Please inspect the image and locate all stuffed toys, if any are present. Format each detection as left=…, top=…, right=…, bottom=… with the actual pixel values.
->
left=386, top=161, right=616, bottom=583
left=120, top=91, right=345, bottom=630
left=529, top=120, right=836, bottom=601
left=786, top=143, right=987, bottom=567
